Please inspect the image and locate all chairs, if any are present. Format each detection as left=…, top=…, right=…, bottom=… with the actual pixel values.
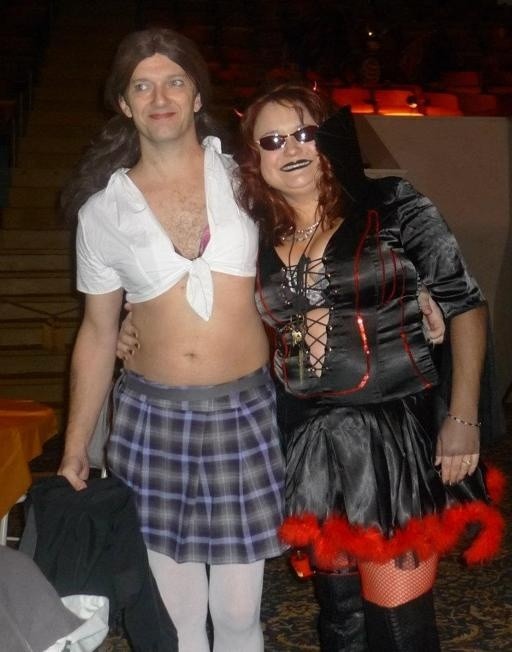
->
left=0, top=0, right=50, bottom=168
left=143, top=0, right=512, bottom=117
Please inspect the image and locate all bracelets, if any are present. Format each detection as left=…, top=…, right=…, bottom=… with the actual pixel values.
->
left=445, top=412, right=483, bottom=430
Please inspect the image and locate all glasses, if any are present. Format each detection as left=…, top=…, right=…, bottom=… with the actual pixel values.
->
left=251, top=124, right=319, bottom=152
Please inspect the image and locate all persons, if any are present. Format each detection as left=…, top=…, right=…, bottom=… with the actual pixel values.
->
left=52, top=25, right=452, bottom=652
left=114, top=76, right=493, bottom=652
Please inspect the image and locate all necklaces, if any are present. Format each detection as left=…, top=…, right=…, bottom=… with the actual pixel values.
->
left=279, top=217, right=326, bottom=350
left=280, top=223, right=320, bottom=243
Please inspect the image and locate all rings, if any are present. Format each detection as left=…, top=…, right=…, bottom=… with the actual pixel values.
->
left=462, top=459, right=473, bottom=465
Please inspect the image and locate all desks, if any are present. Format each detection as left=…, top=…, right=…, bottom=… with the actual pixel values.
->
left=0, top=395, right=59, bottom=547
